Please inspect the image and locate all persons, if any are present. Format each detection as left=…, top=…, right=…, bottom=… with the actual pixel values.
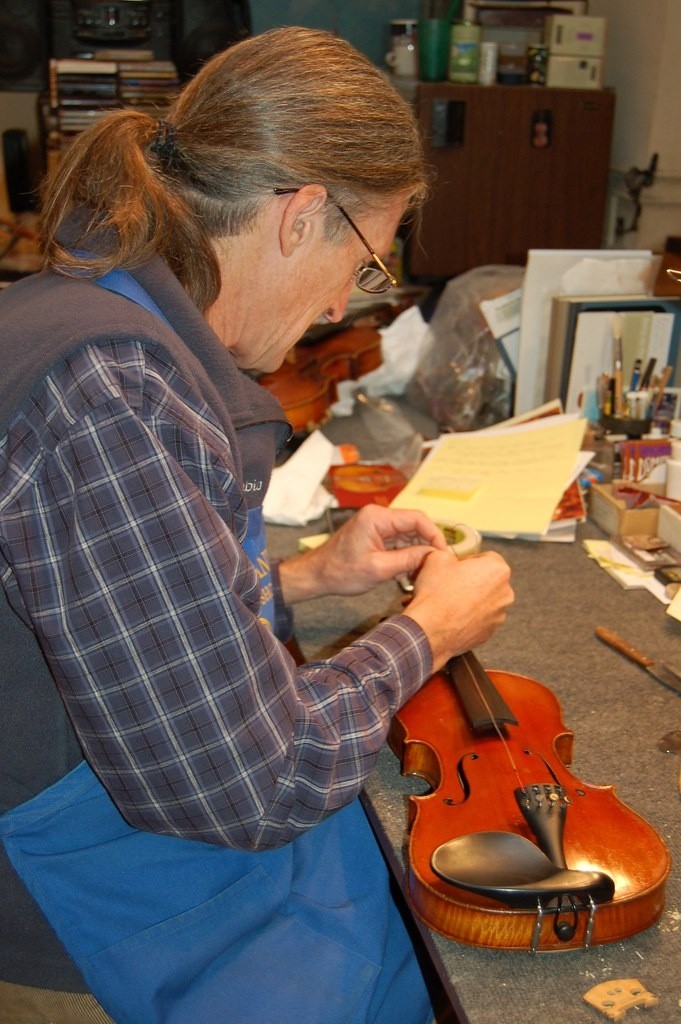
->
left=0, top=24, right=516, bottom=1024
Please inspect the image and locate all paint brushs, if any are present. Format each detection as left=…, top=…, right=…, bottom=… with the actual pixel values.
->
left=596, top=315, right=674, bottom=421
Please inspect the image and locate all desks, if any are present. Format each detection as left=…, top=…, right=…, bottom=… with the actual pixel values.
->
left=228, top=380, right=681, bottom=1024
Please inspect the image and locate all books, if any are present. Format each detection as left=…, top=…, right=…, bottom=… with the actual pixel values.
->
left=543, top=289, right=678, bottom=432
left=386, top=397, right=596, bottom=542
left=55, top=48, right=180, bottom=160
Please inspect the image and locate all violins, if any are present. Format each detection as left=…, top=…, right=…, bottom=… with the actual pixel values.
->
left=390, top=594, right=671, bottom=951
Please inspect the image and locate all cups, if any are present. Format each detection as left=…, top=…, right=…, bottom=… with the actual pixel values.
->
left=420, top=19, right=447, bottom=80
left=599, top=413, right=651, bottom=461
left=387, top=18, right=418, bottom=77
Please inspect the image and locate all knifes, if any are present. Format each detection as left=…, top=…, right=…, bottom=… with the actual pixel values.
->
left=594, top=626, right=681, bottom=693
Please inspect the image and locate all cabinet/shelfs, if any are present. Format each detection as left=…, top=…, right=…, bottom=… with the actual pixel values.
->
left=366, top=66, right=618, bottom=307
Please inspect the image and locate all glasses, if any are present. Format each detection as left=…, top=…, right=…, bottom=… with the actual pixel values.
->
left=273, top=187, right=399, bottom=293
